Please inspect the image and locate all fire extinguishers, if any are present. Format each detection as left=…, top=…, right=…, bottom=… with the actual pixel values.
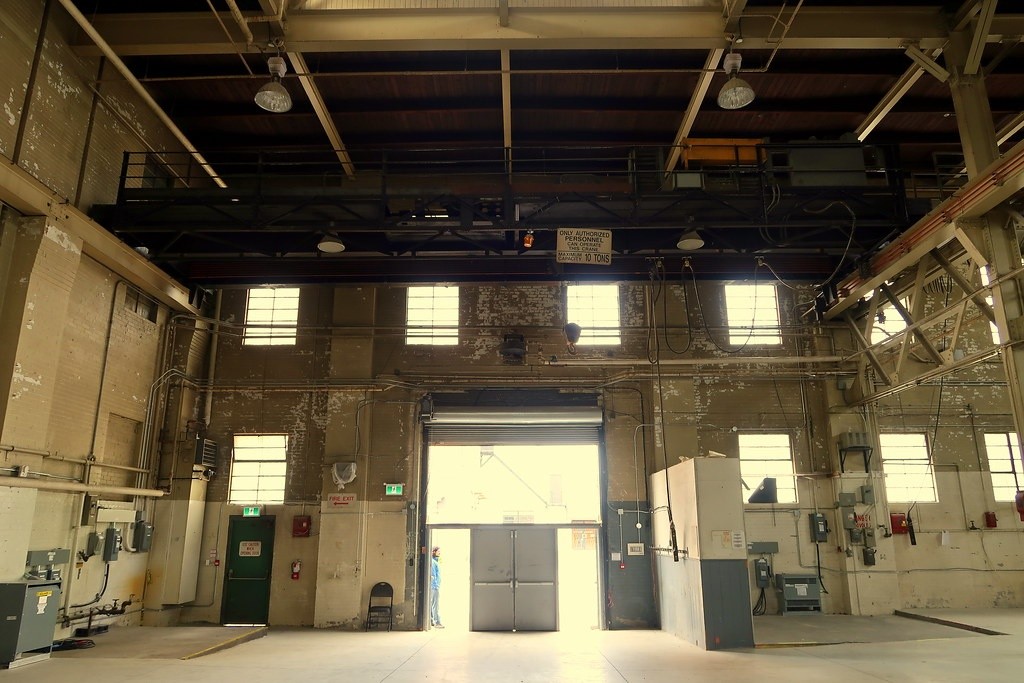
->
left=291, top=560, right=301, bottom=581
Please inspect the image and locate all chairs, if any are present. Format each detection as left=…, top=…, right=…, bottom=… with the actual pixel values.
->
left=365, top=582, right=393, bottom=632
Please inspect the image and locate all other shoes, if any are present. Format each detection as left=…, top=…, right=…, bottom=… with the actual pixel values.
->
left=435, top=624, right=445, bottom=628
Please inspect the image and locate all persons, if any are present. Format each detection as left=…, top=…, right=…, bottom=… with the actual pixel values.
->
left=431, top=546, right=445, bottom=629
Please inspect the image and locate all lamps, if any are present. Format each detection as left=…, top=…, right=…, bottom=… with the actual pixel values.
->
left=717, top=40, right=755, bottom=109
left=317, top=222, right=345, bottom=253
left=254, top=48, right=292, bottom=113
left=677, top=216, right=704, bottom=250
